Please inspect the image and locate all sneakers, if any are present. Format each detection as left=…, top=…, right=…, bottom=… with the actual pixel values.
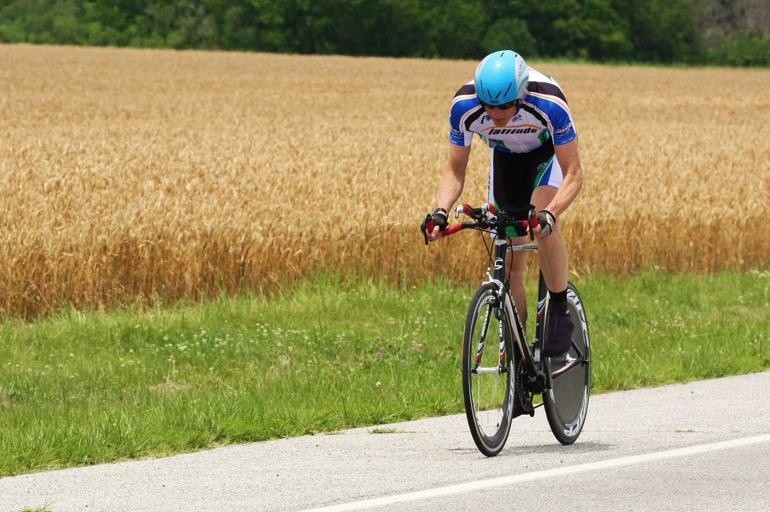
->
left=545, top=311, right=575, bottom=358
left=501, top=369, right=521, bottom=418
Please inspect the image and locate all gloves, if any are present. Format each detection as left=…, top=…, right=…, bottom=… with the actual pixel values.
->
left=420, top=208, right=448, bottom=235
left=532, top=209, right=556, bottom=239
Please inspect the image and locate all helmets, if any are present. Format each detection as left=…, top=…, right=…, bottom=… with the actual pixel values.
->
left=473, top=49, right=528, bottom=105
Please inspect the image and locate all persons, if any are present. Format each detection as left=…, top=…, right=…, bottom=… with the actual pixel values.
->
left=424, top=50, right=584, bottom=357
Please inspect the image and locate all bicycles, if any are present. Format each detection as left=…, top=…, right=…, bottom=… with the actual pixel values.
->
left=425, top=204, right=591, bottom=457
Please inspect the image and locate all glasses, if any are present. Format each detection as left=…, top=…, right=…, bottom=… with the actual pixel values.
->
left=479, top=98, right=521, bottom=110
